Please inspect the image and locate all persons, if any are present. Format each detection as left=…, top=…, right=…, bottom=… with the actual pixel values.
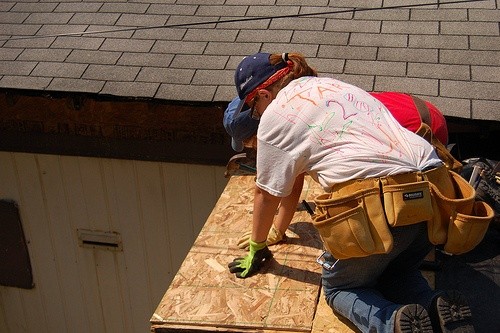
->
left=223, top=91, right=448, bottom=251
left=228, top=52, right=494, bottom=333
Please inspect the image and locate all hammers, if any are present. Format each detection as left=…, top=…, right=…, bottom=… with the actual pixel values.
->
left=224, top=153, right=257, bottom=179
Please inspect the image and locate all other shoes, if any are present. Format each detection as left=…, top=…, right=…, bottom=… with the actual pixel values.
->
left=437, top=293, right=475, bottom=333
left=395, top=303, right=434, bottom=332
left=476, top=179, right=500, bottom=213
left=477, top=159, right=500, bottom=189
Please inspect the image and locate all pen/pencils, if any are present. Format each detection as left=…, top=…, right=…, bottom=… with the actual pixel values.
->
left=302, top=198, right=315, bottom=215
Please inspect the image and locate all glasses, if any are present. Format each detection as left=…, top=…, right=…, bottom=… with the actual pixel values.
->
left=247, top=96, right=259, bottom=118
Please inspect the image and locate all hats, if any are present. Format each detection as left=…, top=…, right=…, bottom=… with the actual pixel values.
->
left=231, top=51, right=287, bottom=118
left=223, top=94, right=259, bottom=152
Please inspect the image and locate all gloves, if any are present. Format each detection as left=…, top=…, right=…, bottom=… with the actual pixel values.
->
left=236, top=221, right=283, bottom=251
left=228, top=236, right=272, bottom=277
